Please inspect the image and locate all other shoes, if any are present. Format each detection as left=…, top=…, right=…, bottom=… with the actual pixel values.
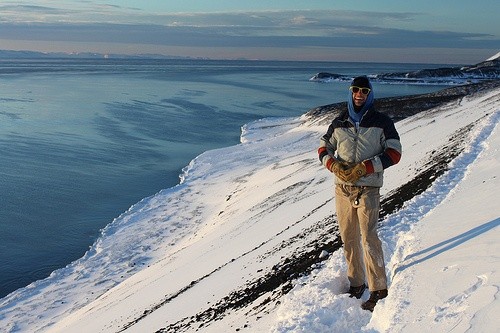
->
left=346, top=282, right=367, bottom=300
left=362, top=288, right=388, bottom=312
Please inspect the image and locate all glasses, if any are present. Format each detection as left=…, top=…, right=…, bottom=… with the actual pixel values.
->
left=350, top=86, right=372, bottom=95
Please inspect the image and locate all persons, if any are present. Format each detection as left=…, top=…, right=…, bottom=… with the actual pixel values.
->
left=318, top=76, right=402, bottom=312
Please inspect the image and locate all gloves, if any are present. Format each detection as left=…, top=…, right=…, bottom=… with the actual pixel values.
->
left=332, top=160, right=350, bottom=181
left=344, top=162, right=366, bottom=185
